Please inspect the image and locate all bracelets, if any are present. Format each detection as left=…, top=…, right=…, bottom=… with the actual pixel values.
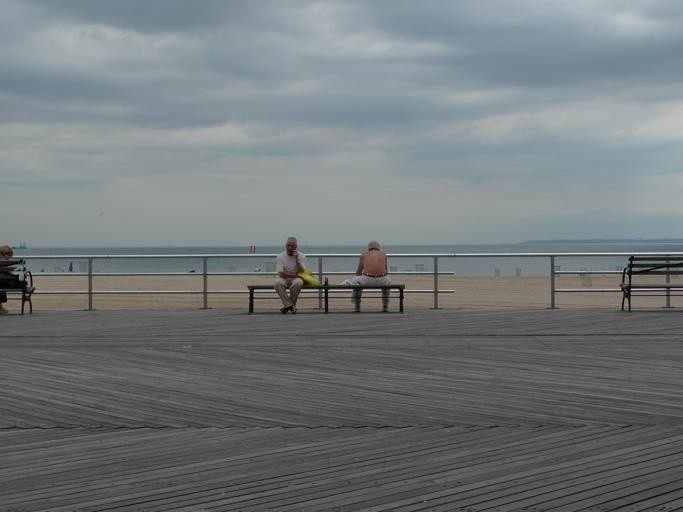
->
left=296, top=260, right=302, bottom=264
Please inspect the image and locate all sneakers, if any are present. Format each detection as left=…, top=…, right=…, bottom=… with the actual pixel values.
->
left=382, top=309, right=389, bottom=312
left=280, top=305, right=298, bottom=314
left=1, top=310, right=7, bottom=313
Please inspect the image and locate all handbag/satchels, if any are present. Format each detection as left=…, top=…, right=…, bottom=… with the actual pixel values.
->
left=298, top=268, right=322, bottom=286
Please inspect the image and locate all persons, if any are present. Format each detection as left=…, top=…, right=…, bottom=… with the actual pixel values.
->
left=350, top=240, right=392, bottom=313
left=0, top=245, right=29, bottom=314
left=273, top=236, right=306, bottom=313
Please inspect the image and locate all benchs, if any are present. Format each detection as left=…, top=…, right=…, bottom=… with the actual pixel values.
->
left=619, top=254, right=683, bottom=313
left=247, top=279, right=406, bottom=313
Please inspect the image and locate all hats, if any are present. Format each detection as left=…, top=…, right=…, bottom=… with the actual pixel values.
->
left=368, top=241, right=380, bottom=250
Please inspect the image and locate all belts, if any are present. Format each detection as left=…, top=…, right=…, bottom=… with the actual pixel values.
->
left=363, top=273, right=387, bottom=277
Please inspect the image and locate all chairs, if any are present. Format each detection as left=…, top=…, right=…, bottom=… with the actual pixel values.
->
left=0, top=259, right=35, bottom=315
